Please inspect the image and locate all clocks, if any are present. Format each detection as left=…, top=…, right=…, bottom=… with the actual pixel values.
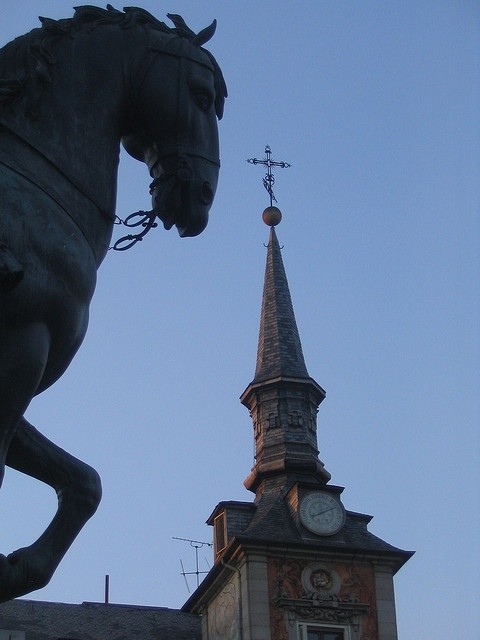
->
left=299, top=490, right=347, bottom=537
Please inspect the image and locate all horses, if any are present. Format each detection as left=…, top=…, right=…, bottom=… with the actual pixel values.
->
left=2, top=4, right=228, bottom=603
